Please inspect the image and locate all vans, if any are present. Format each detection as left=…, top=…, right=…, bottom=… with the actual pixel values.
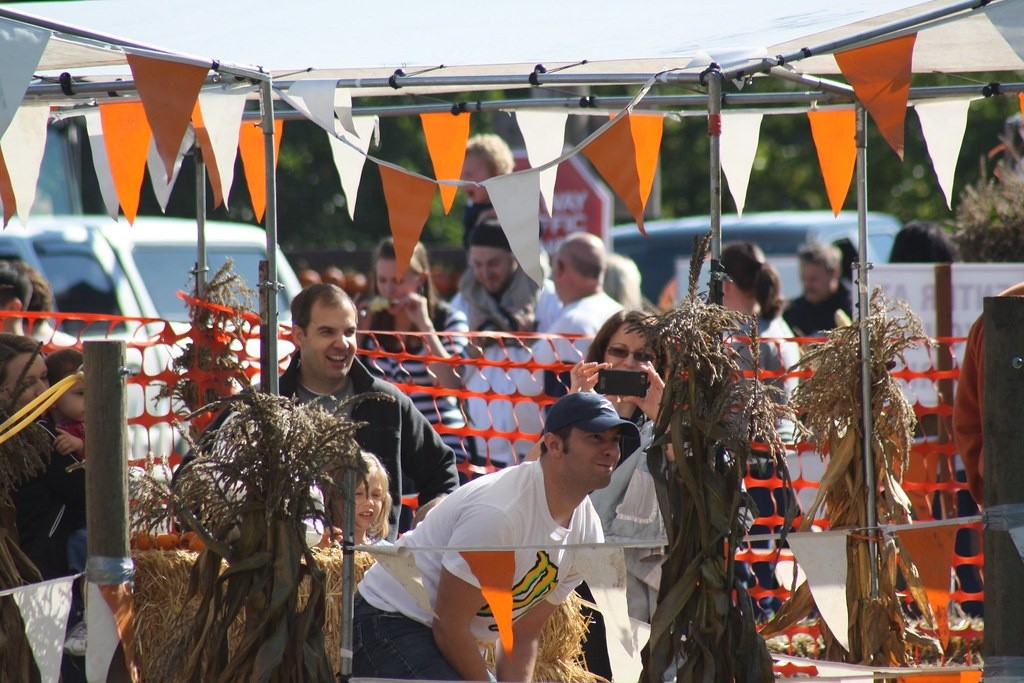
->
left=0, top=216, right=307, bottom=505
left=613, top=210, right=903, bottom=312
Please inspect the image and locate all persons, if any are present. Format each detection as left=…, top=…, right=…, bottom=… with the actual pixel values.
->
left=0, top=260, right=85, bottom=682
left=354, top=393, right=642, bottom=682
left=718, top=221, right=984, bottom=623
left=169, top=283, right=460, bottom=549
left=524, top=310, right=671, bottom=621
left=358, top=132, right=644, bottom=479
left=950, top=283, right=1024, bottom=516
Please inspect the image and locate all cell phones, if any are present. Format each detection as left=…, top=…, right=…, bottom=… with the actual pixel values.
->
left=598, top=369, right=648, bottom=398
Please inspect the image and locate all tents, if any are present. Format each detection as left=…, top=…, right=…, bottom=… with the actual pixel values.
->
left=0, top=0, right=1024, bottom=683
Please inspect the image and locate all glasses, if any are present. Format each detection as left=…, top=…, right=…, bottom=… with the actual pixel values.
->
left=603, top=346, right=658, bottom=363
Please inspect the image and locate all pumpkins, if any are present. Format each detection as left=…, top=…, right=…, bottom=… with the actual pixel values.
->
left=137, top=529, right=206, bottom=552
left=297, top=265, right=463, bottom=295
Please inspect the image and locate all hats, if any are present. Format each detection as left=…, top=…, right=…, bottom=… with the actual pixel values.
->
left=542, top=391, right=640, bottom=437
left=470, top=215, right=544, bottom=249
